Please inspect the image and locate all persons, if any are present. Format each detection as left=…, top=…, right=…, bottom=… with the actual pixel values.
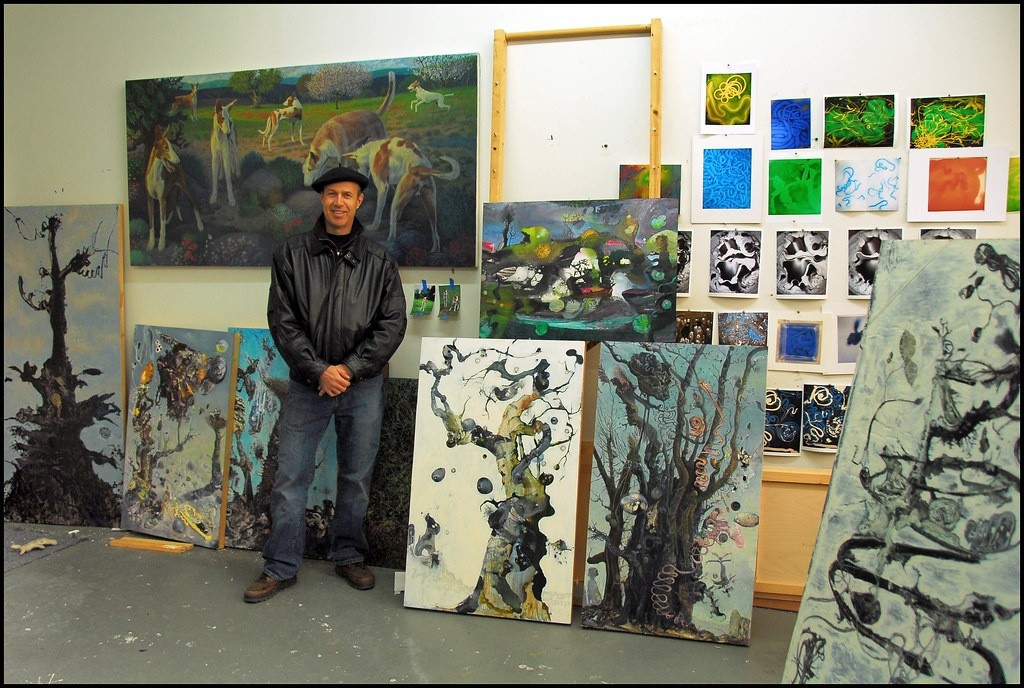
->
left=243, top=165, right=407, bottom=602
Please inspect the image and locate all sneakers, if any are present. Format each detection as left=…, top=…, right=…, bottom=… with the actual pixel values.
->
left=335, top=559, right=375, bottom=589
left=244, top=572, right=297, bottom=603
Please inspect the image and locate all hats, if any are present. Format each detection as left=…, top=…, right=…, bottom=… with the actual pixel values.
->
left=311, top=167, right=369, bottom=193
